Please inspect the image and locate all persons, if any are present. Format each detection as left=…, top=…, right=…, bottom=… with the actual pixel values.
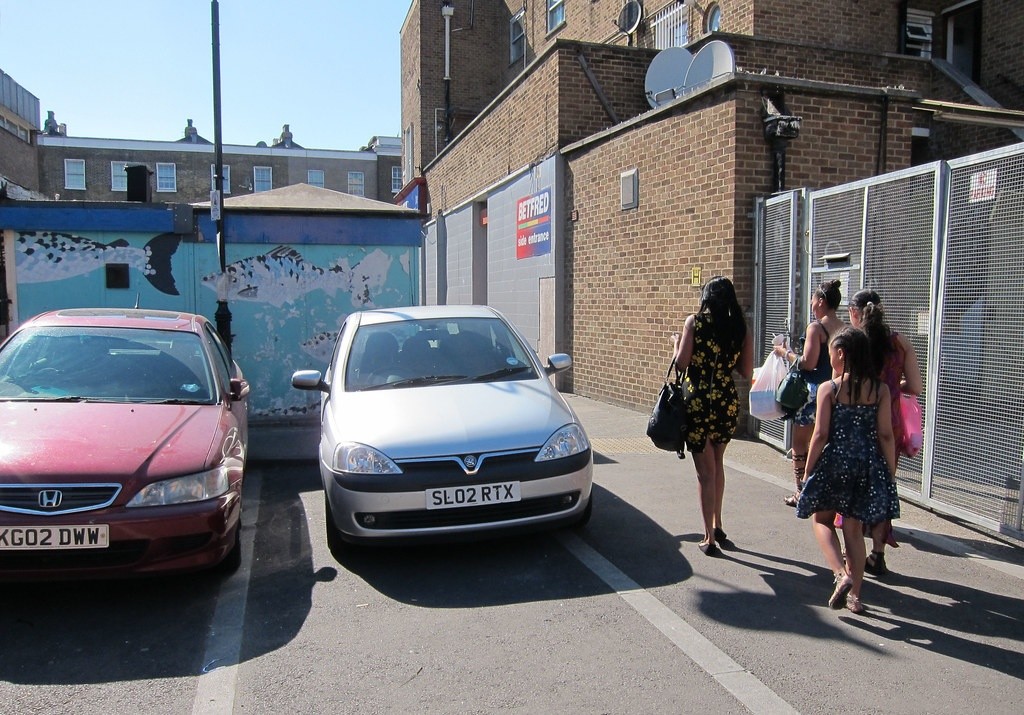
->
left=801, top=327, right=897, bottom=612
left=833, top=286, right=923, bottom=574
left=771, top=280, right=845, bottom=506
left=671, top=277, right=753, bottom=556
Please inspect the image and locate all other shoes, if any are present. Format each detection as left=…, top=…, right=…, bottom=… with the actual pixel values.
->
left=698, top=540, right=716, bottom=557
left=783, top=454, right=806, bottom=508
left=714, top=529, right=726, bottom=542
left=847, top=594, right=862, bottom=612
left=828, top=577, right=854, bottom=609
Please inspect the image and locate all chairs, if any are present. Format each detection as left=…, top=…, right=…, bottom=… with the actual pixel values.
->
left=358, top=331, right=485, bottom=378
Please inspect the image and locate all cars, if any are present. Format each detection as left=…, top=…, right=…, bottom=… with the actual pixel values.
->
left=291, top=305, right=594, bottom=553
left=0, top=307, right=250, bottom=583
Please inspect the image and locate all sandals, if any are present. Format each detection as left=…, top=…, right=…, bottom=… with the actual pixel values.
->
left=841, top=548, right=846, bottom=566
left=863, top=551, right=888, bottom=575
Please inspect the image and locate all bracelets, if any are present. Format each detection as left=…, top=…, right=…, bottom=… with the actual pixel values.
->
left=786, top=350, right=791, bottom=361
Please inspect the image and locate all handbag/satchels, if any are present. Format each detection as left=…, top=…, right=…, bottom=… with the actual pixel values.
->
left=893, top=388, right=923, bottom=457
left=748, top=349, right=789, bottom=422
left=647, top=356, right=687, bottom=459
left=776, top=358, right=819, bottom=410
